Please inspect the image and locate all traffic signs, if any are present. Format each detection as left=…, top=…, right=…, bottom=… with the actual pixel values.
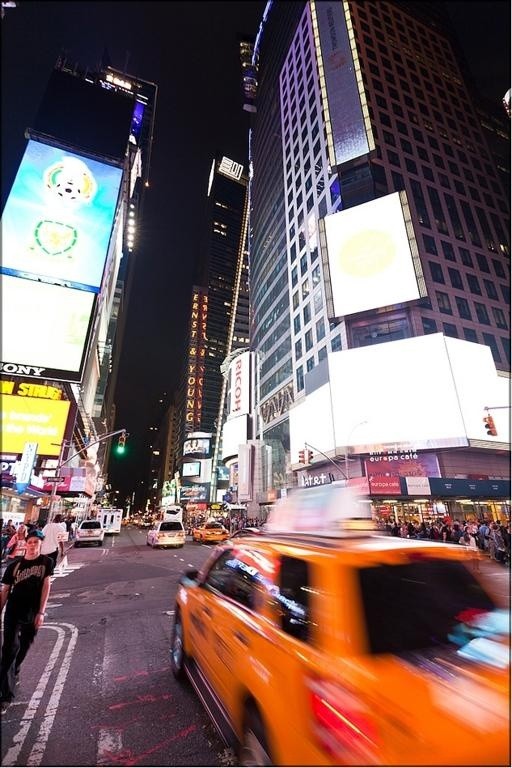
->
left=47, top=476, right=65, bottom=481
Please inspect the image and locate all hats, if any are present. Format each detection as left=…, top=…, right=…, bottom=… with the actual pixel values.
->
left=24, top=529, right=45, bottom=542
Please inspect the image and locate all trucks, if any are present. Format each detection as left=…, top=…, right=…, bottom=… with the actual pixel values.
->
left=157, top=504, right=184, bottom=523
left=96, top=508, right=124, bottom=534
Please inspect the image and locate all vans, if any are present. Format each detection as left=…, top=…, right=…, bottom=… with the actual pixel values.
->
left=145, top=521, right=186, bottom=548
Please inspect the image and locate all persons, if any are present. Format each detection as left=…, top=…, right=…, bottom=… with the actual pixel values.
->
left=375, top=515, right=510, bottom=574
left=184, top=513, right=267, bottom=538
left=1, top=529, right=54, bottom=712
left=0, top=513, right=75, bottom=570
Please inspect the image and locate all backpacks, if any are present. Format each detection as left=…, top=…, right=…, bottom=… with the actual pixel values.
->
left=484, top=527, right=491, bottom=540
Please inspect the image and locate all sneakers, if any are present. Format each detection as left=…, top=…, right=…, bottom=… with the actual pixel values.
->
left=9, top=669, right=22, bottom=688
left=0, top=697, right=17, bottom=716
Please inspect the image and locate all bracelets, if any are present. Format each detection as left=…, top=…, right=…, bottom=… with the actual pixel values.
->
left=38, top=611, right=45, bottom=615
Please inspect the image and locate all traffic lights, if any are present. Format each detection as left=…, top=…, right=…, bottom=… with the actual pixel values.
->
left=116, top=435, right=126, bottom=454
left=307, top=449, right=313, bottom=464
left=298, top=449, right=305, bottom=463
left=482, top=415, right=498, bottom=437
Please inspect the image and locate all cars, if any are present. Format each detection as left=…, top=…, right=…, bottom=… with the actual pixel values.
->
left=191, top=521, right=231, bottom=544
left=74, top=519, right=105, bottom=548
left=168, top=532, right=511, bottom=766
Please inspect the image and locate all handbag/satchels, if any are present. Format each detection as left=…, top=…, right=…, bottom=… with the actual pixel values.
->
left=5, top=535, right=17, bottom=555
